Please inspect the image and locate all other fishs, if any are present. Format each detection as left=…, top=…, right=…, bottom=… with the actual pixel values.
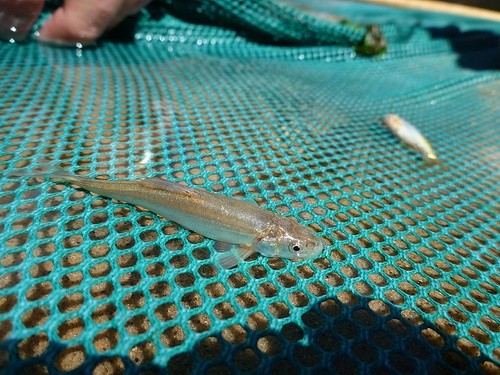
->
left=9, top=158, right=324, bottom=270
left=383, top=113, right=441, bottom=163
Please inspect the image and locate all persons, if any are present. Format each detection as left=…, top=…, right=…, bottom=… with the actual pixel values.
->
left=0, top=1, right=153, bottom=49
